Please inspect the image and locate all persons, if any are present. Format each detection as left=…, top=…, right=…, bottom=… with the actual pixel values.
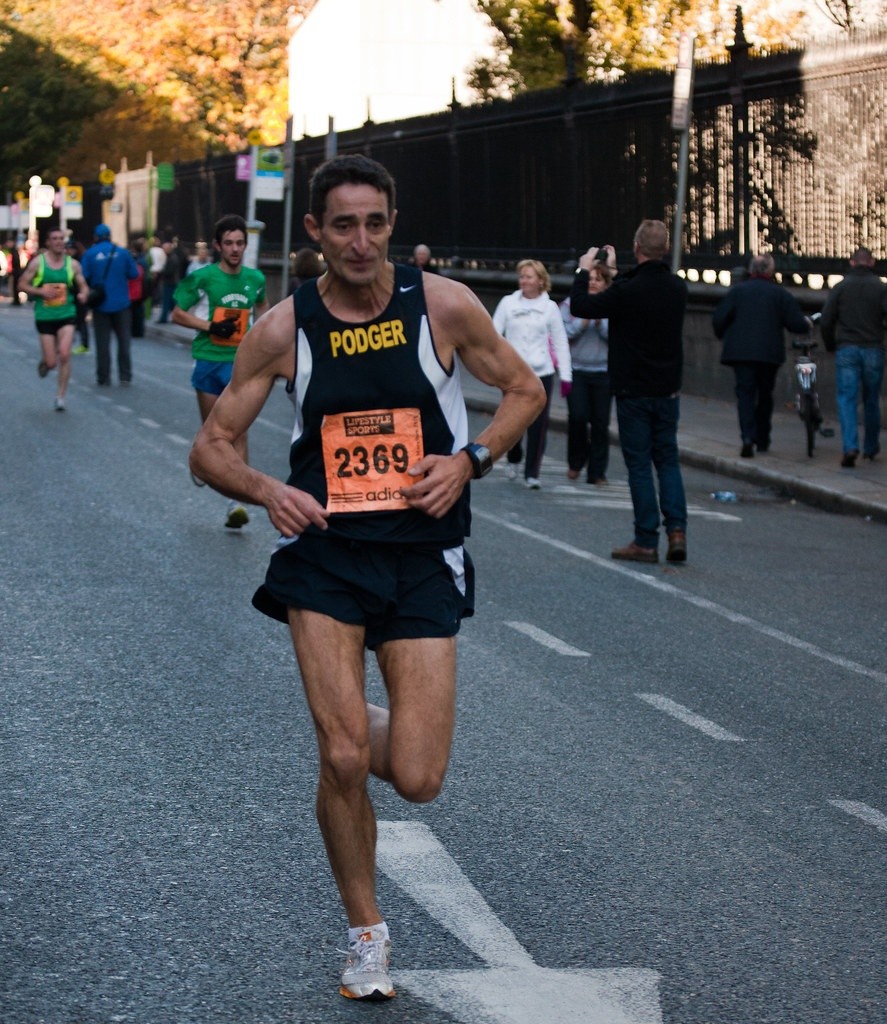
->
left=414, top=244, right=438, bottom=275
left=82, top=224, right=138, bottom=387
left=64, top=240, right=90, bottom=355
left=186, top=247, right=211, bottom=276
left=128, top=236, right=181, bottom=339
left=173, top=214, right=269, bottom=529
left=190, top=155, right=548, bottom=998
left=820, top=247, right=887, bottom=467
left=492, top=260, right=573, bottom=490
left=571, top=220, right=688, bottom=564
left=294, top=248, right=320, bottom=283
left=713, top=254, right=813, bottom=459
left=559, top=264, right=613, bottom=487
left=17, top=227, right=89, bottom=411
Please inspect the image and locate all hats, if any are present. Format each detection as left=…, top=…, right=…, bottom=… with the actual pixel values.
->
left=95, top=224, right=111, bottom=236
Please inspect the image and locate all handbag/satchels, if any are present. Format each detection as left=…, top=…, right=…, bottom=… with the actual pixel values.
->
left=82, top=284, right=106, bottom=311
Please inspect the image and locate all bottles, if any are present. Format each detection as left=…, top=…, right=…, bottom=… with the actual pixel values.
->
left=709, top=489, right=736, bottom=503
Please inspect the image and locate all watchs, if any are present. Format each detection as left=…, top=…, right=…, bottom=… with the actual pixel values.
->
left=460, top=443, right=492, bottom=479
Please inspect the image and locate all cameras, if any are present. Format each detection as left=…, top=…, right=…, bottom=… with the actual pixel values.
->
left=596, top=249, right=609, bottom=261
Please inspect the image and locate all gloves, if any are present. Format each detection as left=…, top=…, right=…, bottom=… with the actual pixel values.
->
left=207, top=314, right=239, bottom=340
left=560, top=381, right=571, bottom=397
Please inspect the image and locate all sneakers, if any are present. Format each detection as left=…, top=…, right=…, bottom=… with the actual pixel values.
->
left=666, top=528, right=686, bottom=561
left=612, top=541, right=658, bottom=564
left=335, top=928, right=396, bottom=999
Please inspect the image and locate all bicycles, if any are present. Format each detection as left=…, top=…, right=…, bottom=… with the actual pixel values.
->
left=786, top=311, right=838, bottom=458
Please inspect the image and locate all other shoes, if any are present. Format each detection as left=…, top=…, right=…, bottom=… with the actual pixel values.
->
left=863, top=443, right=880, bottom=459
left=54, top=397, right=65, bottom=411
left=740, top=438, right=754, bottom=459
left=223, top=499, right=249, bottom=529
left=841, top=450, right=857, bottom=469
left=525, top=477, right=542, bottom=488
left=567, top=469, right=579, bottom=478
left=73, top=345, right=92, bottom=354
left=504, top=462, right=519, bottom=479
left=756, top=440, right=769, bottom=453
left=598, top=478, right=609, bottom=486
left=39, top=361, right=48, bottom=378
